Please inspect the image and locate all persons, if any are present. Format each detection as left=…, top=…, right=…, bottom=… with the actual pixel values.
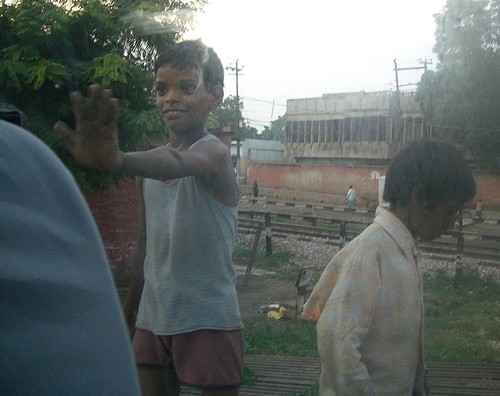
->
left=252, top=180, right=259, bottom=203
left=475, top=200, right=484, bottom=218
left=53, top=40, right=245, bottom=396
left=300, top=139, right=477, bottom=396
left=0, top=118, right=142, bottom=396
left=345, top=185, right=356, bottom=209
